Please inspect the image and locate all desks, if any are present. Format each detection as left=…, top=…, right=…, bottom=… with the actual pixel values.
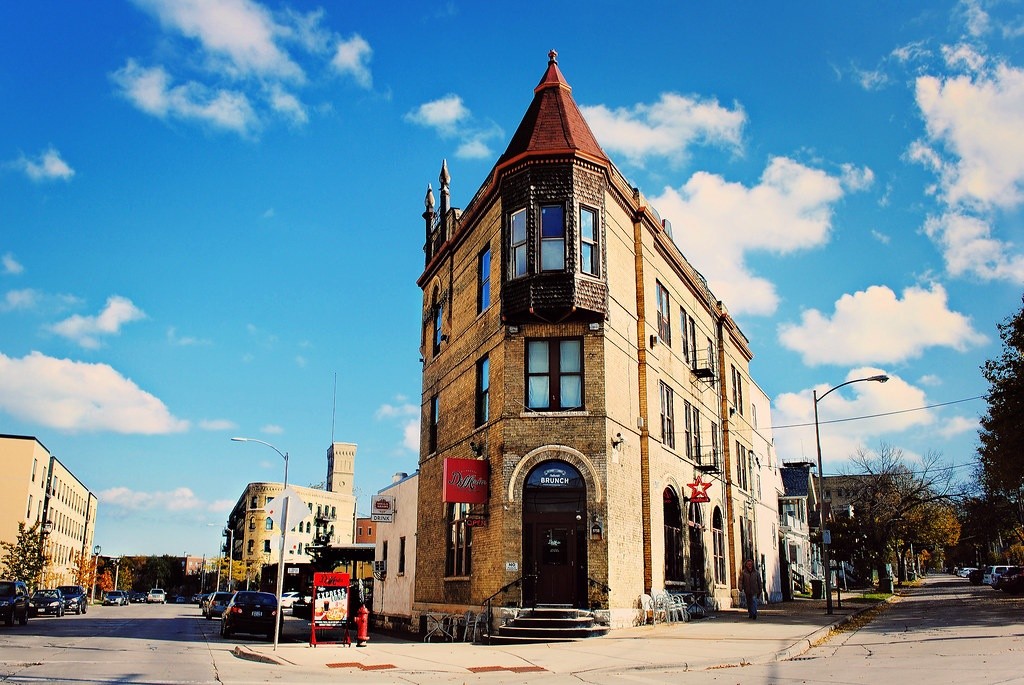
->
left=683, top=590, right=707, bottom=620
left=424, top=613, right=453, bottom=642
left=670, top=593, right=693, bottom=620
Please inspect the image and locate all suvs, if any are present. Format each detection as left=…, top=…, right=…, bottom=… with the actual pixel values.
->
left=984, top=565, right=1018, bottom=588
left=0, top=580, right=31, bottom=626
left=146, top=589, right=168, bottom=604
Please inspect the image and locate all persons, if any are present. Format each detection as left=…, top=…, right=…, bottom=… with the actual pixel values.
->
left=739, top=560, right=763, bottom=620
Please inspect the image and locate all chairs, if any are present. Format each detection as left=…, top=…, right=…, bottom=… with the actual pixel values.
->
left=640, top=588, right=688, bottom=626
left=463, top=608, right=488, bottom=642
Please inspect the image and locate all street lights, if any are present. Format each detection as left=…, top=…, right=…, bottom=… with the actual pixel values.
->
left=39, top=520, right=52, bottom=587
left=812, top=375, right=889, bottom=613
left=114, top=557, right=123, bottom=592
left=88, top=544, right=101, bottom=605
left=231, top=437, right=289, bottom=654
left=208, top=523, right=233, bottom=592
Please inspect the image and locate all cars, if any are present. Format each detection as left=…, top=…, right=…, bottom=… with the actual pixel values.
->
left=122, top=590, right=131, bottom=604
left=998, top=567, right=1024, bottom=593
left=280, top=590, right=312, bottom=609
left=103, top=590, right=125, bottom=606
left=190, top=591, right=234, bottom=620
left=220, top=591, right=285, bottom=640
left=941, top=565, right=984, bottom=585
left=56, top=585, right=89, bottom=615
left=28, top=589, right=66, bottom=617
left=176, top=597, right=186, bottom=604
left=132, top=593, right=147, bottom=603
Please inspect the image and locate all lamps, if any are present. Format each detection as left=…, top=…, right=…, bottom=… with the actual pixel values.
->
left=589, top=323, right=599, bottom=330
left=441, top=334, right=448, bottom=341
left=420, top=359, right=425, bottom=363
left=509, top=326, right=518, bottom=333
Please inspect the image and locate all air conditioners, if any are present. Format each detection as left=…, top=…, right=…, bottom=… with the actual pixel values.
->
left=372, top=560, right=386, bottom=572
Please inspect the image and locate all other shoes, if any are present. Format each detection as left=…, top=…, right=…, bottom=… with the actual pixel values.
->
left=752, top=615, right=758, bottom=620
left=748, top=614, right=752, bottom=618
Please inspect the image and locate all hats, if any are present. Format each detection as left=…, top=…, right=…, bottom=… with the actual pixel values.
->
left=746, top=558, right=754, bottom=563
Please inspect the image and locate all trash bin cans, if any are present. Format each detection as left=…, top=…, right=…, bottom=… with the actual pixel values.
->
left=809, top=580, right=825, bottom=599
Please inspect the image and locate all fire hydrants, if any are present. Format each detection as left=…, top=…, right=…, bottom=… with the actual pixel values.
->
left=353, top=606, right=374, bottom=647
